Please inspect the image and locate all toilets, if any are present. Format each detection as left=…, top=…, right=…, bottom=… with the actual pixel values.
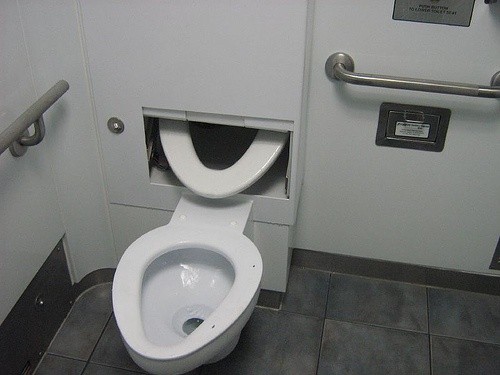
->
left=112, top=118, right=290, bottom=375
